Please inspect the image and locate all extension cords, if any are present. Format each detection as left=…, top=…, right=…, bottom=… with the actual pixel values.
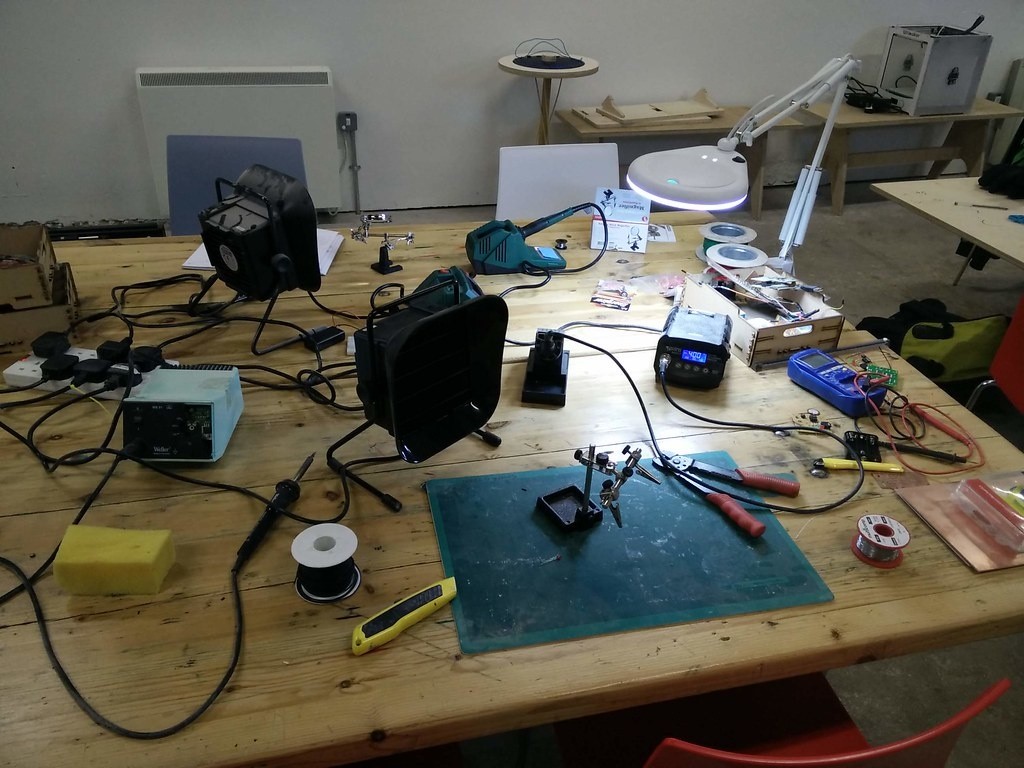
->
left=4, top=346, right=179, bottom=401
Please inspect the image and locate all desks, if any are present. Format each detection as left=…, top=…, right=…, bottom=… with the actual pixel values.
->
left=871, top=176, right=1024, bottom=286
left=0, top=210, right=1024, bottom=768
left=498, top=54, right=598, bottom=144
left=789, top=97, right=1024, bottom=216
left=555, top=106, right=804, bottom=220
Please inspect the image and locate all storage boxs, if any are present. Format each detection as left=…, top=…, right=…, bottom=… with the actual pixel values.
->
left=681, top=265, right=844, bottom=367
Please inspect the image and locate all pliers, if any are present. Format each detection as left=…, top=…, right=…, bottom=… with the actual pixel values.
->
left=652, top=450, right=801, bottom=538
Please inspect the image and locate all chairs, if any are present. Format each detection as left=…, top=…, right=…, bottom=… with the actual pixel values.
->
left=516, top=673, right=1011, bottom=768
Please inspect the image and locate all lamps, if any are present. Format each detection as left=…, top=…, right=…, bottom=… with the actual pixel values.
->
left=626, top=51, right=862, bottom=276
